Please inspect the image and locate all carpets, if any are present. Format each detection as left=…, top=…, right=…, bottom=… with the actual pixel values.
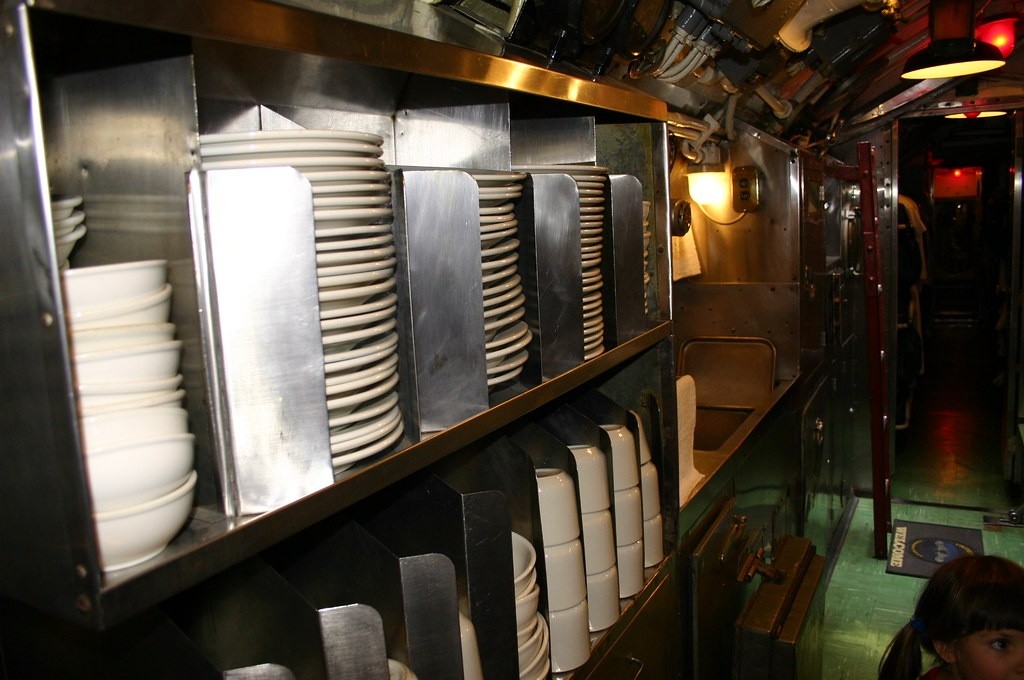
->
left=885, top=519, right=984, bottom=579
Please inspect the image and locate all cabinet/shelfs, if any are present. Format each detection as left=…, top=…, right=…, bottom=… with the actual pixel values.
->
left=0, top=0, right=684, bottom=680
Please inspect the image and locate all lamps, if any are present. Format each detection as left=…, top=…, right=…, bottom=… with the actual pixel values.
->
left=900, top=0, right=1008, bottom=81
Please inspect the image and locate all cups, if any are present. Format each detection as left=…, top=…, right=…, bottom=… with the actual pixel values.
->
left=535, top=469, right=592, bottom=673
left=625, top=409, right=666, bottom=567
left=565, top=446, right=620, bottom=631
left=596, top=425, right=643, bottom=599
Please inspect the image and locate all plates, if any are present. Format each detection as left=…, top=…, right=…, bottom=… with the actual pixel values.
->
left=565, top=175, right=608, bottom=361
left=199, top=127, right=403, bottom=475
left=467, top=173, right=531, bottom=392
left=511, top=164, right=611, bottom=174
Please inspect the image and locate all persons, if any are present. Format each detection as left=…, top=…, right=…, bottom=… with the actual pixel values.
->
left=876, top=554, right=1024, bottom=679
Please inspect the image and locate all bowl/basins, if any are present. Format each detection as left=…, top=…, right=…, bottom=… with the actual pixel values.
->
left=382, top=532, right=550, bottom=680
left=47, top=195, right=198, bottom=573
